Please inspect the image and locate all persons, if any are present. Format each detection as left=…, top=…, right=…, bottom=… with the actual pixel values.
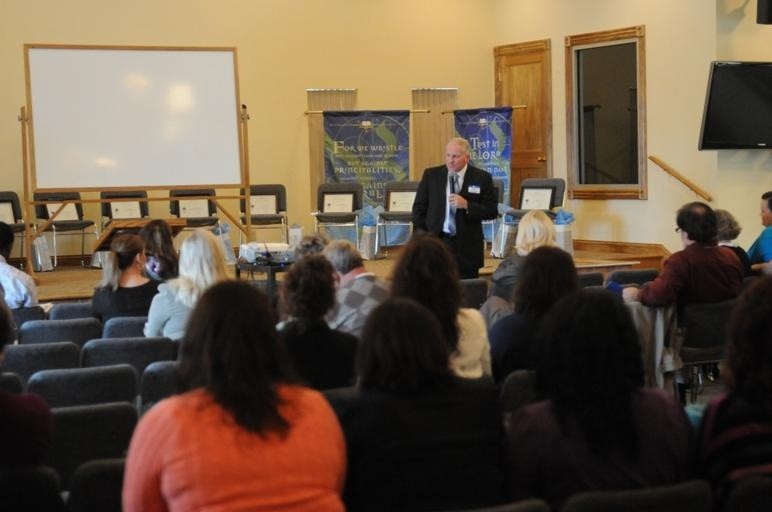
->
left=714, top=209, right=752, bottom=277
left=143, top=229, right=230, bottom=340
left=506, top=287, right=705, bottom=510
left=122, top=279, right=347, bottom=511
left=391, top=237, right=491, bottom=378
left=621, top=202, right=744, bottom=405
left=90, top=235, right=164, bottom=325
left=699, top=274, right=771, bottom=469
left=2, top=221, right=38, bottom=309
left=412, top=139, right=498, bottom=279
left=333, top=296, right=508, bottom=508
left=1, top=392, right=50, bottom=510
left=493, top=209, right=560, bottom=288
left=489, top=245, right=580, bottom=386
left=1, top=284, right=17, bottom=345
left=277, top=255, right=360, bottom=391
left=746, top=192, right=770, bottom=263
left=294, top=231, right=331, bottom=262
left=323, top=240, right=392, bottom=337
left=138, top=219, right=179, bottom=282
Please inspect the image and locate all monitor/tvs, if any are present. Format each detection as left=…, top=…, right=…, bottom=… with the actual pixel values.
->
left=697, top=58, right=772, bottom=150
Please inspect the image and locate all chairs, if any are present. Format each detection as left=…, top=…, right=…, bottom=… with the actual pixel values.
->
left=481, top=179, right=505, bottom=247
left=100, top=190, right=148, bottom=231
left=169, top=190, right=219, bottom=239
left=311, top=180, right=364, bottom=251
left=33, top=192, right=94, bottom=268
left=0, top=268, right=772, bottom=512
left=374, top=180, right=421, bottom=260
left=0, top=191, right=26, bottom=270
left=499, top=177, right=565, bottom=259
left=240, top=185, right=289, bottom=244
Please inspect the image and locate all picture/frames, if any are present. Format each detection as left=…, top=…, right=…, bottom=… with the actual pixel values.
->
left=564, top=24, right=646, bottom=201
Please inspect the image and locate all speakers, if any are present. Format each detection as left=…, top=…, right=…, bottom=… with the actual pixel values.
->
left=754, top=0, right=772, bottom=24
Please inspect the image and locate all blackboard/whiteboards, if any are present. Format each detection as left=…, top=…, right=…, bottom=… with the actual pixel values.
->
left=23, top=42, right=244, bottom=193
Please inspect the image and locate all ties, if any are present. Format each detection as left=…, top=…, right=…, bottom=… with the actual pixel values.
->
left=449, top=174, right=459, bottom=236
